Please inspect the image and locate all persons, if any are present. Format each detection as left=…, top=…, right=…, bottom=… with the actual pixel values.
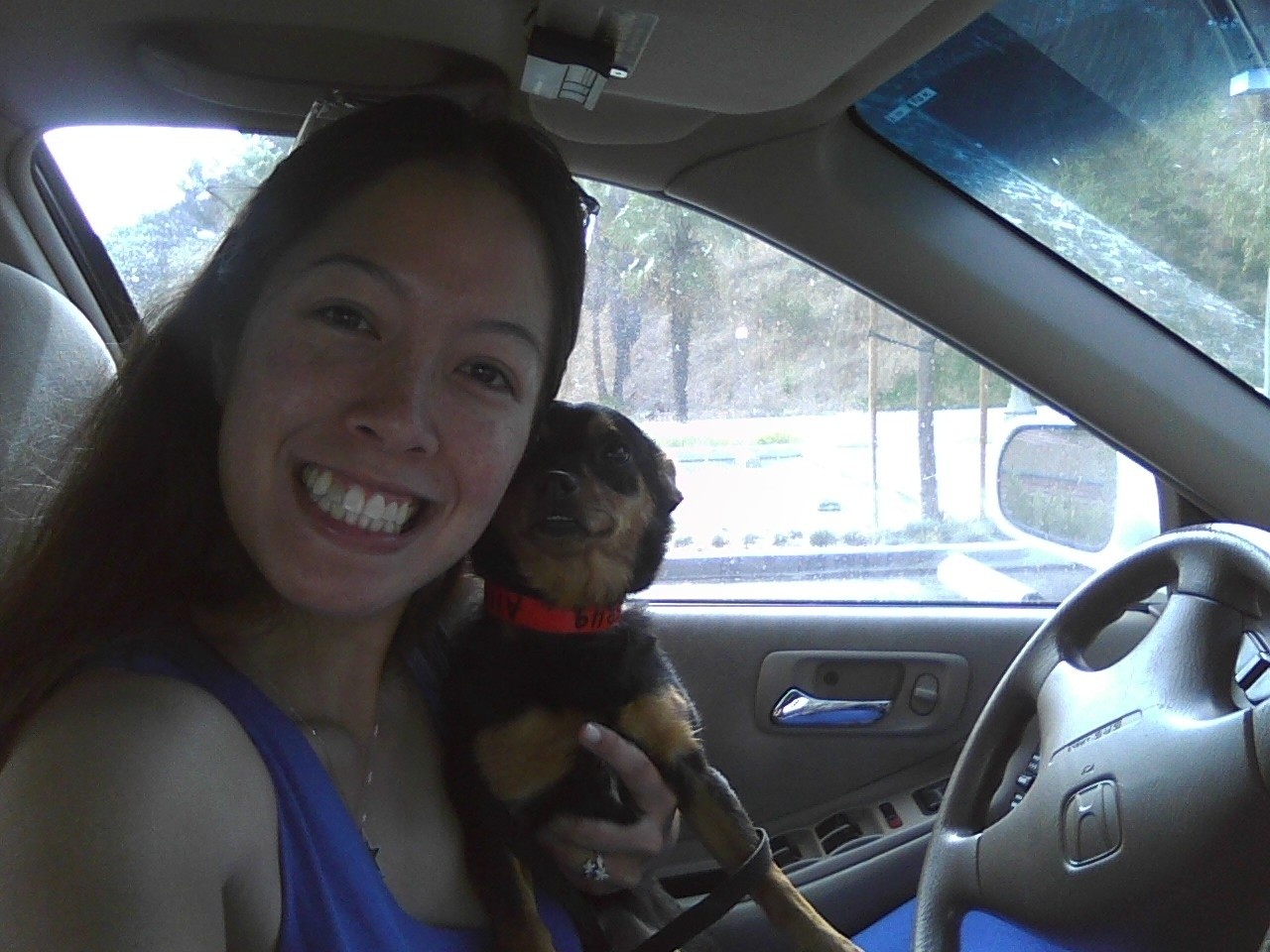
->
left=1, top=92, right=1080, bottom=952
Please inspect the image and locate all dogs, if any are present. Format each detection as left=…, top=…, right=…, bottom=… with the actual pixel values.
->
left=435, top=399, right=865, bottom=952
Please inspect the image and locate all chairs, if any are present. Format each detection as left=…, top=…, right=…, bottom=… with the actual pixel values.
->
left=1, top=264, right=121, bottom=579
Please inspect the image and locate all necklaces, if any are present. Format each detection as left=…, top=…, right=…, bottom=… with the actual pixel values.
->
left=353, top=665, right=393, bottom=878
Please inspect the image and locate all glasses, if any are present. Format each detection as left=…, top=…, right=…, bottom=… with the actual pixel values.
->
left=288, top=86, right=601, bottom=232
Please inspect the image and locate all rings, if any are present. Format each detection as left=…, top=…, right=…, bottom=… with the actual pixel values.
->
left=582, top=854, right=608, bottom=883
